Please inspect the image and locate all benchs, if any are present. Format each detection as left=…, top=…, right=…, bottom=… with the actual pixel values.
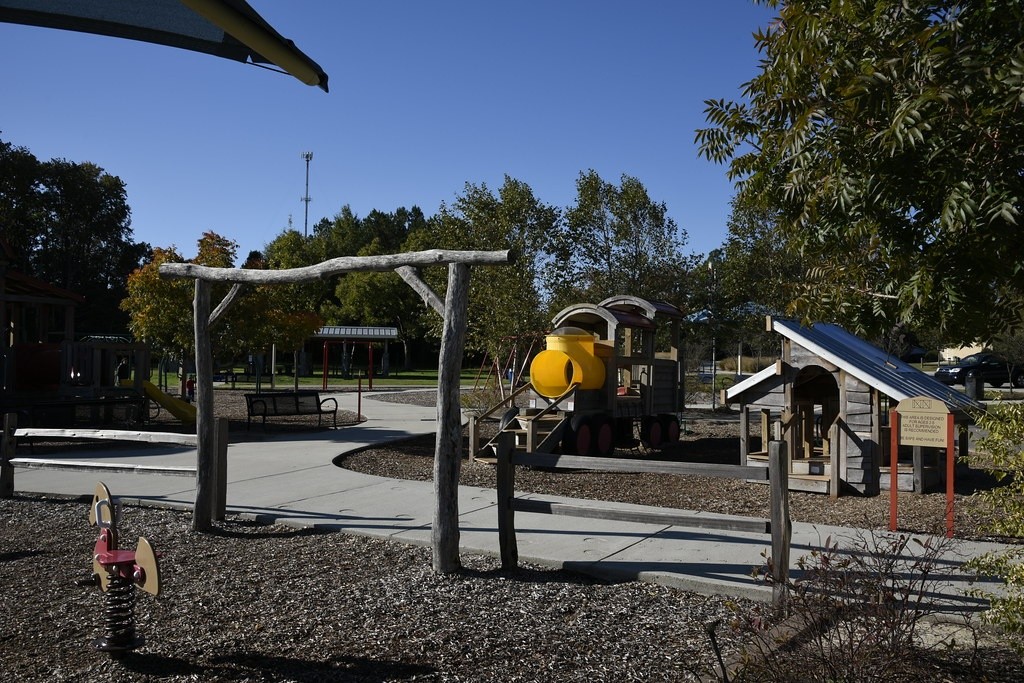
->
left=234, top=373, right=274, bottom=383
left=213, top=373, right=234, bottom=382
left=244, top=390, right=339, bottom=434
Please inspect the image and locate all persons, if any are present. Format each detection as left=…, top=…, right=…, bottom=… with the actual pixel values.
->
left=187, top=374, right=196, bottom=402
left=116, top=357, right=129, bottom=383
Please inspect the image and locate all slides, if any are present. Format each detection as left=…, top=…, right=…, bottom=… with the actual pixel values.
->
left=143, top=380, right=196, bottom=420
